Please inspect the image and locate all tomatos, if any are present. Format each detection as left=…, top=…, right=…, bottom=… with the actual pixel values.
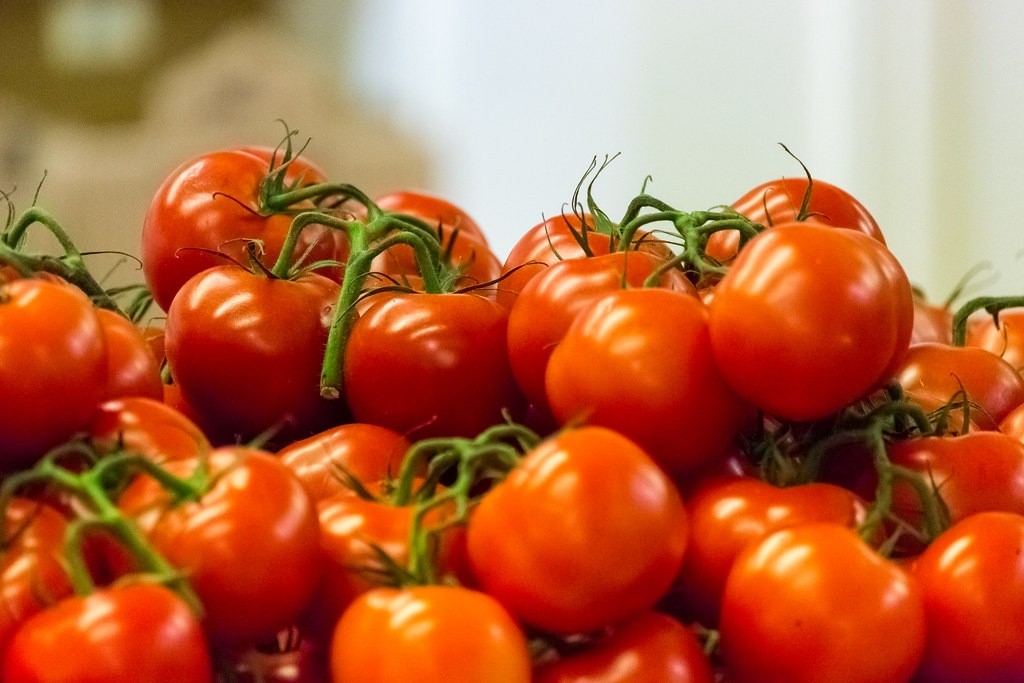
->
left=0, top=114, right=1023, bottom=683
